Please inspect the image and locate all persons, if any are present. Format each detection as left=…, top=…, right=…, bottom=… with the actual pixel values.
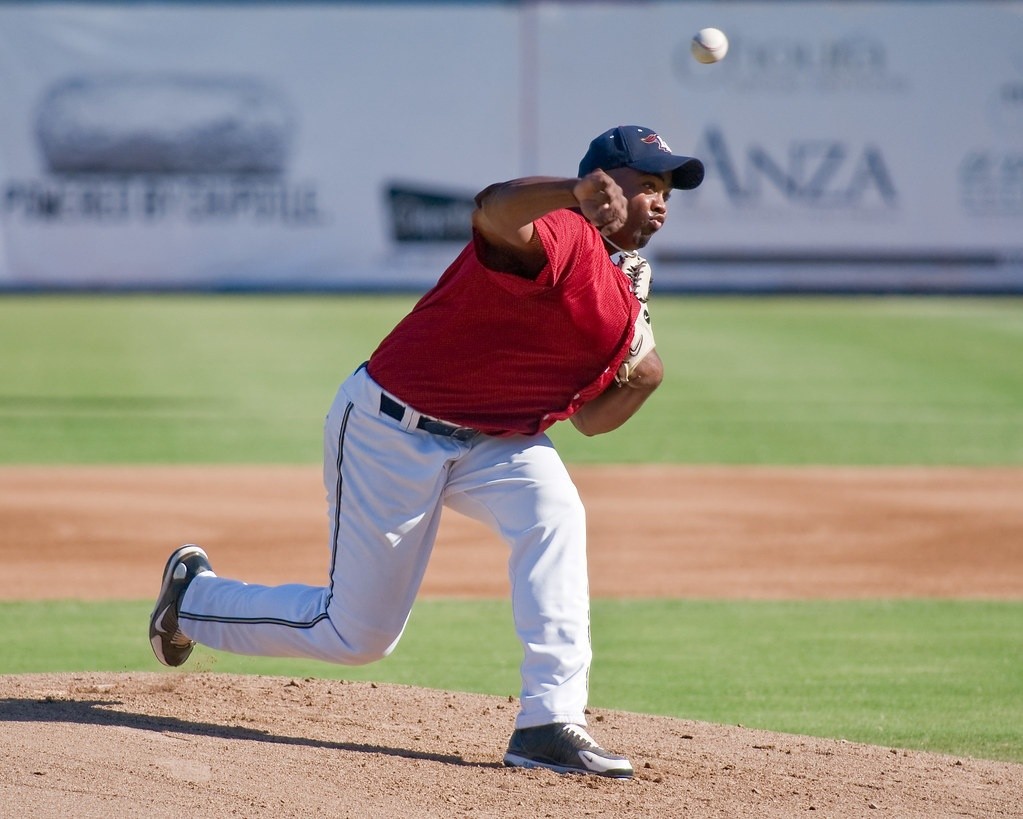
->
left=149, top=124, right=705, bottom=781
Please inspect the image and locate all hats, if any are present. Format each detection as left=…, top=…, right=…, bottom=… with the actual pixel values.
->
left=578, top=125, right=704, bottom=190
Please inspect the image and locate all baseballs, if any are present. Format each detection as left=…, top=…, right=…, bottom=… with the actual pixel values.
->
left=691, top=28, right=730, bottom=64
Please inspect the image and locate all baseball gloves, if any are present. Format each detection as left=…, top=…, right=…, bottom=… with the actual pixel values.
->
left=605, top=249, right=658, bottom=386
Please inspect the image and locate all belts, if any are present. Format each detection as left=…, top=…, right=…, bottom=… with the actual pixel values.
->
left=380, top=394, right=482, bottom=442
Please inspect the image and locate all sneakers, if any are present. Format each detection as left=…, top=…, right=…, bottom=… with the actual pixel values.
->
left=148, top=544, right=213, bottom=668
left=504, top=722, right=634, bottom=780
